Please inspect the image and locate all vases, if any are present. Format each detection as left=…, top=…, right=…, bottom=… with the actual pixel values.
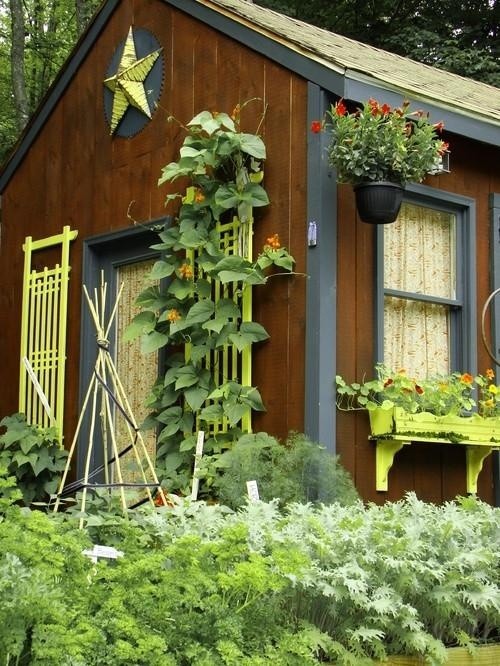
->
left=355, top=171, right=404, bottom=225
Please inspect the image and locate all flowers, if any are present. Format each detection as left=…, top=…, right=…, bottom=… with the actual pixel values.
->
left=309, top=98, right=450, bottom=182
left=338, top=363, right=500, bottom=418
